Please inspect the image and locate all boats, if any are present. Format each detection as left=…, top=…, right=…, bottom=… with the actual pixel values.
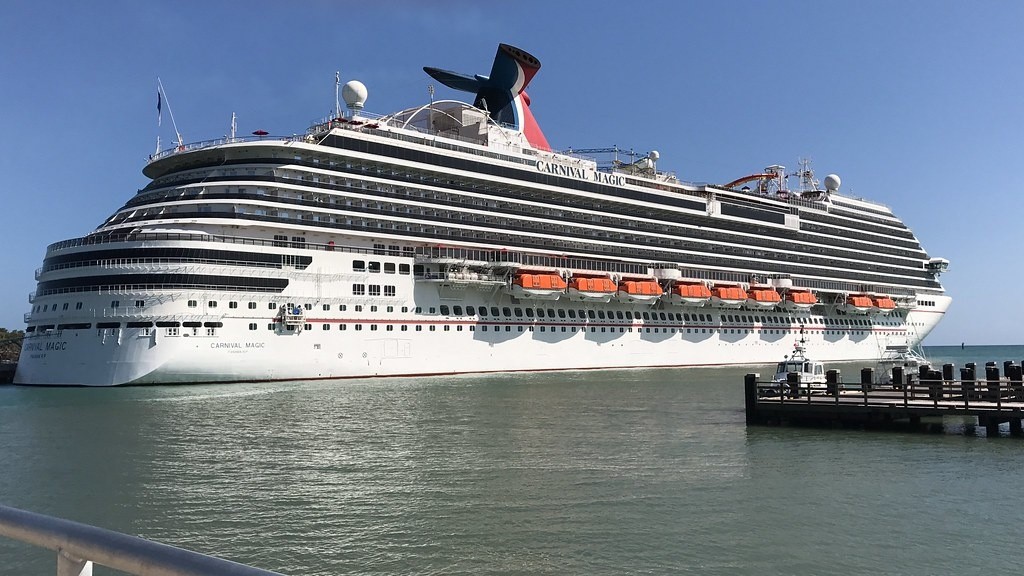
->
left=710, top=287, right=748, bottom=308
left=746, top=289, right=782, bottom=311
left=670, top=282, right=710, bottom=308
left=759, top=322, right=867, bottom=398
left=875, top=297, right=895, bottom=312
left=782, top=293, right=817, bottom=310
left=618, top=280, right=660, bottom=305
left=869, top=333, right=938, bottom=388
left=509, top=272, right=565, bottom=299
left=843, top=296, right=871, bottom=313
left=569, top=276, right=618, bottom=306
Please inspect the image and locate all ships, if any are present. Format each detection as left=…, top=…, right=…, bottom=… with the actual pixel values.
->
left=10, top=42, right=954, bottom=389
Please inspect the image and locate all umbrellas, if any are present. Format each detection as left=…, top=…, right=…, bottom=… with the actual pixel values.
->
left=252, top=130, right=269, bottom=140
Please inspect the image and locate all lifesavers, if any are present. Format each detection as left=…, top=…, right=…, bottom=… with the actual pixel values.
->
left=178, top=145, right=184, bottom=151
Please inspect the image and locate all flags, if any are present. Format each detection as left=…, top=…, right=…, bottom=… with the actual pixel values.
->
left=156, top=86, right=162, bottom=126
